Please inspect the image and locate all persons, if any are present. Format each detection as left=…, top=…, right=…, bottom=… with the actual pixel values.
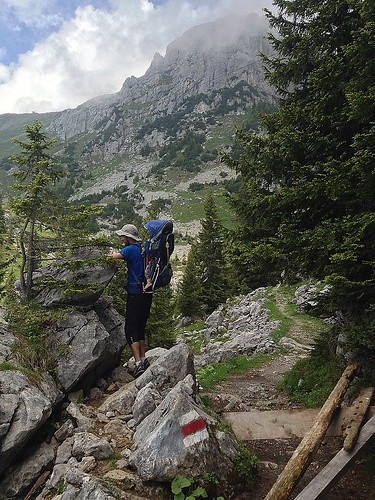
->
left=107, top=224, right=153, bottom=378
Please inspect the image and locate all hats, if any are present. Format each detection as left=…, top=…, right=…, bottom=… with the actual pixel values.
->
left=115, top=224, right=142, bottom=242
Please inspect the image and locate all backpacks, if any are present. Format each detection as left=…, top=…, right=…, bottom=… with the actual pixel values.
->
left=133, top=219, right=175, bottom=293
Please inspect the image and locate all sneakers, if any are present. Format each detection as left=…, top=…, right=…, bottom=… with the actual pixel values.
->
left=128, top=359, right=150, bottom=374
left=133, top=361, right=144, bottom=377
